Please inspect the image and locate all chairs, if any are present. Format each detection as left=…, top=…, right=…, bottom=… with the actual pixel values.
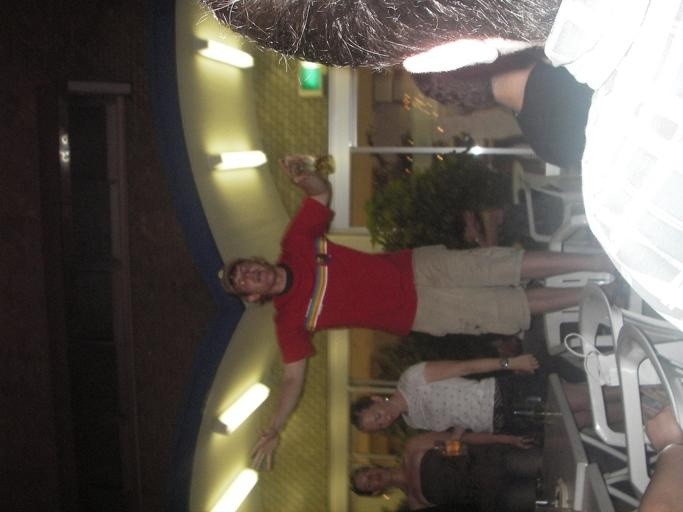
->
left=511, top=160, right=683, bottom=512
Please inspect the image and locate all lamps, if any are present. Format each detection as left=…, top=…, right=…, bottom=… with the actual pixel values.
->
left=192, top=35, right=256, bottom=70
left=212, top=382, right=270, bottom=435
left=207, top=149, right=268, bottom=170
left=208, top=467, right=259, bottom=512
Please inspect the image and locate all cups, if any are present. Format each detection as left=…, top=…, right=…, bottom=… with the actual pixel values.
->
left=430, top=440, right=463, bottom=456
left=285, top=156, right=339, bottom=178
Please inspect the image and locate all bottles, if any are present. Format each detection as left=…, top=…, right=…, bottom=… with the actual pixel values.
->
left=513, top=404, right=566, bottom=427
